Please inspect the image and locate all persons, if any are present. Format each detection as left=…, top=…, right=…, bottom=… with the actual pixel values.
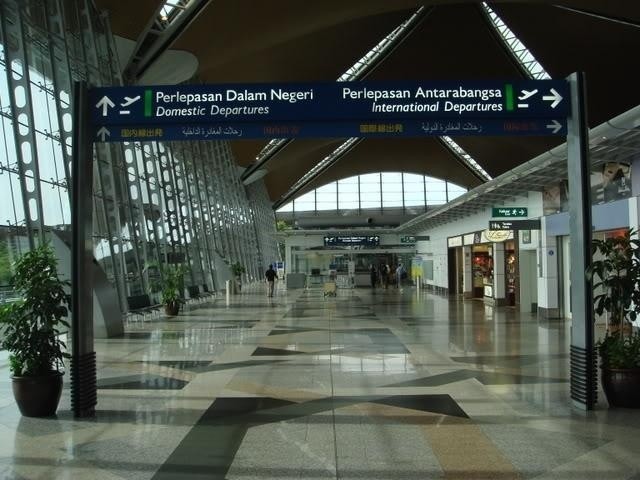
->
left=369, top=263, right=405, bottom=289
left=265, top=264, right=278, bottom=298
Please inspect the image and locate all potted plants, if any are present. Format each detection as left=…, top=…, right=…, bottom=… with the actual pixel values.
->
left=231, top=261, right=246, bottom=293
left=138, top=261, right=190, bottom=316
left=586, top=223, right=640, bottom=409
left=0, top=235, right=70, bottom=417
